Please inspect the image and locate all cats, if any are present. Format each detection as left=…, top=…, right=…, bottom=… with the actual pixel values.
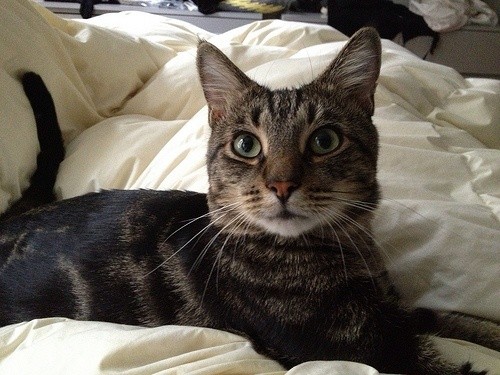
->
left=0, top=26, right=499, bottom=375
